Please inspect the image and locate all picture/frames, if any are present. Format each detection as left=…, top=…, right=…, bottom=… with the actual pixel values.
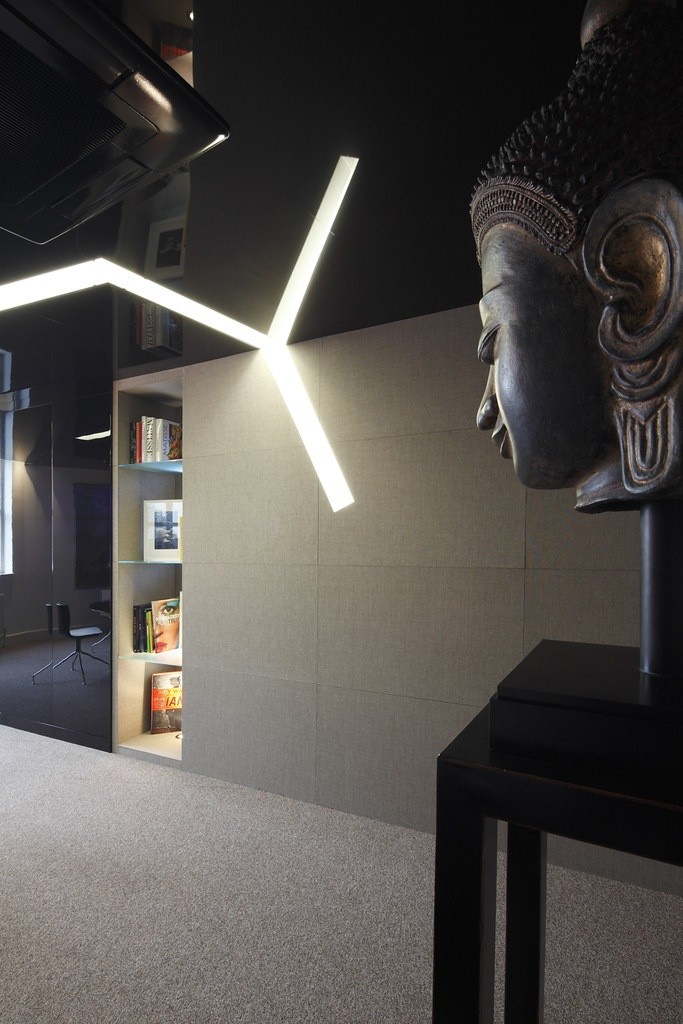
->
left=142, top=499, right=183, bottom=561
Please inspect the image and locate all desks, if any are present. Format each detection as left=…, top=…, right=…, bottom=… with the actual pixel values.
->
left=433, top=638, right=683, bottom=1024
left=89, top=602, right=110, bottom=654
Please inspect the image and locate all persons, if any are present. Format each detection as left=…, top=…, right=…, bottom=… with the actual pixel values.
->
left=469, top=0, right=683, bottom=514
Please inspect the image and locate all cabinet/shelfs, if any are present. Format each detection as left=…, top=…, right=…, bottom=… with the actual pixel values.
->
left=119, top=458, right=182, bottom=668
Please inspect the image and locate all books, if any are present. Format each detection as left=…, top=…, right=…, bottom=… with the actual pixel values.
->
left=129, top=302, right=187, bottom=354
left=129, top=415, right=184, bottom=735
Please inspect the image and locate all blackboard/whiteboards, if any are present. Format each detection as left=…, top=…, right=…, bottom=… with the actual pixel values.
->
left=75, top=482, right=112, bottom=590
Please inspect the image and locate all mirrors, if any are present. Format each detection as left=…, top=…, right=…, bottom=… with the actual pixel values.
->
left=0, top=394, right=113, bottom=752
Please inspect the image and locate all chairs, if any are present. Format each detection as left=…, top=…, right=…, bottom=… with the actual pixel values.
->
left=30, top=602, right=110, bottom=685
